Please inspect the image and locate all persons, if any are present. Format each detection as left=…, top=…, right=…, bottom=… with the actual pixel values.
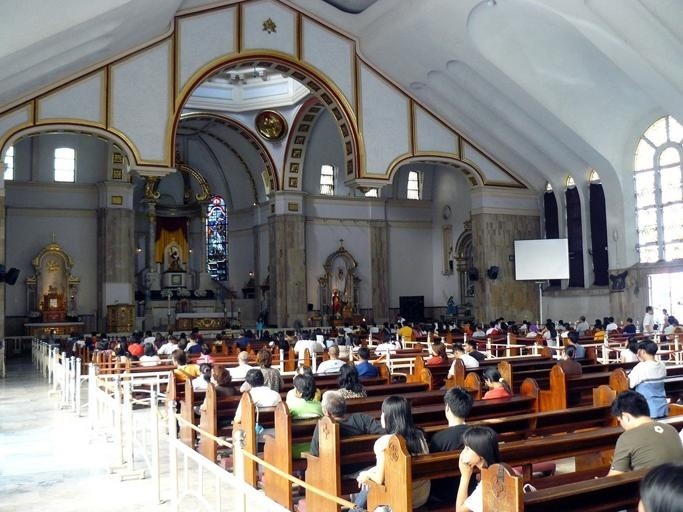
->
left=333, top=292, right=342, bottom=320
left=171, top=251, right=179, bottom=271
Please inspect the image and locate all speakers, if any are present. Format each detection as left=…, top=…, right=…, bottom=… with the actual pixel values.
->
left=5, top=267, right=20, bottom=286
left=468, top=267, right=479, bottom=281
left=489, top=265, right=499, bottom=279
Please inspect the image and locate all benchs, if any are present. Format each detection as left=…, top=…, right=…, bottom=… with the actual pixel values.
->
left=166, top=357, right=683, bottom=512
left=43, top=331, right=683, bottom=408
